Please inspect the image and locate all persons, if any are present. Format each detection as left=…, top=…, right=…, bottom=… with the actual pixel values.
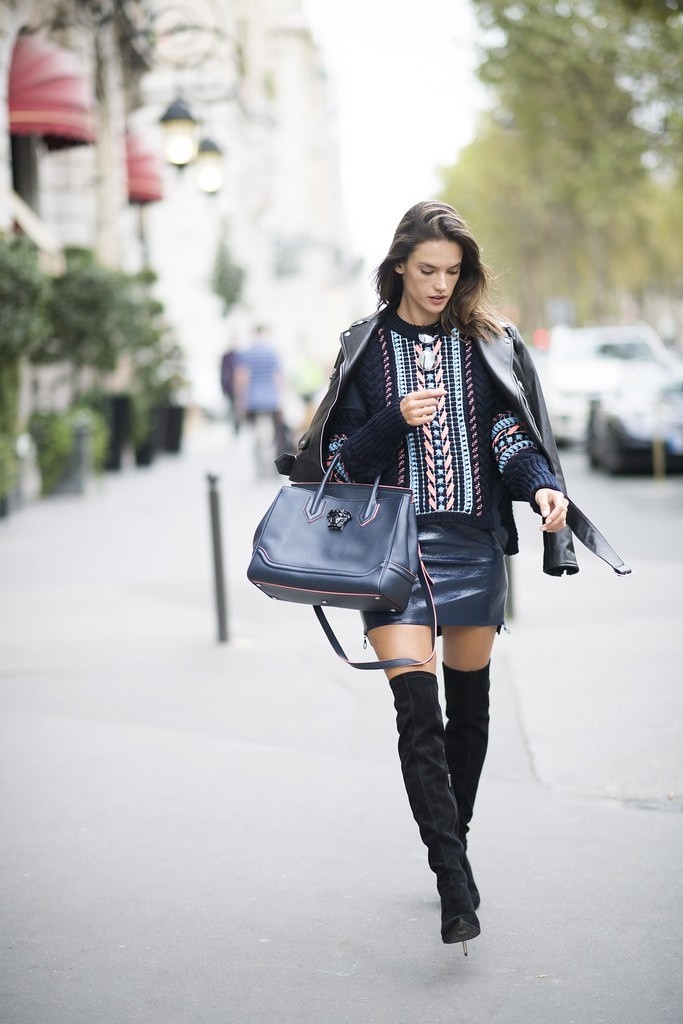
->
left=275, top=203, right=569, bottom=956
left=220, top=326, right=297, bottom=467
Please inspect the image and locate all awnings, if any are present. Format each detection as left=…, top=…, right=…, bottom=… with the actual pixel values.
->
left=127, top=135, right=162, bottom=205
left=9, top=28, right=104, bottom=149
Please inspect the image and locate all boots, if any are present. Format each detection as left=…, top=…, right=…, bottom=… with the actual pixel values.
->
left=442, top=659, right=490, bottom=912
left=389, top=670, right=481, bottom=955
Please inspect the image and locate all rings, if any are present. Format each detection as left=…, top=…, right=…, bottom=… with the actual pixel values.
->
left=563, top=507, right=568, bottom=514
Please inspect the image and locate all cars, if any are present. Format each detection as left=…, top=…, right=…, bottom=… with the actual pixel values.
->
left=522, top=321, right=681, bottom=450
left=583, top=357, right=683, bottom=475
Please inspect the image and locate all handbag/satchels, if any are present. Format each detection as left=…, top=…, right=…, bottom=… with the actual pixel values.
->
left=247, top=450, right=419, bottom=615
